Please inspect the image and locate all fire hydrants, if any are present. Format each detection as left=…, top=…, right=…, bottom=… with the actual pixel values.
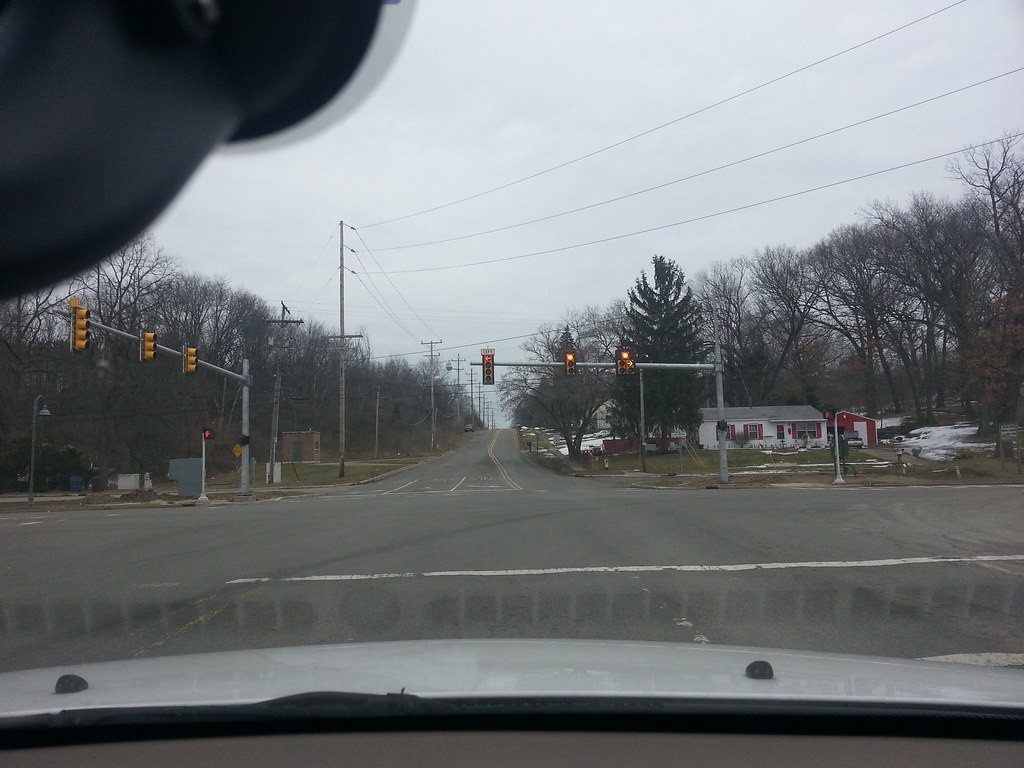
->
left=603, top=458, right=610, bottom=470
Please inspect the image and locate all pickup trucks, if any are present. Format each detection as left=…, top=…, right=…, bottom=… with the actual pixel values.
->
left=844, top=430, right=863, bottom=450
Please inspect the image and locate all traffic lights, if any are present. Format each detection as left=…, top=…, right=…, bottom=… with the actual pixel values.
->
left=564, top=353, right=577, bottom=376
left=203, top=428, right=215, bottom=440
left=186, top=347, right=198, bottom=373
left=75, top=307, right=90, bottom=351
left=482, top=354, right=494, bottom=385
left=615, top=349, right=636, bottom=376
left=822, top=409, right=834, bottom=420
left=144, top=331, right=157, bottom=360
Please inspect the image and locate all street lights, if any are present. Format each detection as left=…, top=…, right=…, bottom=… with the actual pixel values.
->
left=28, top=394, right=51, bottom=504
left=429, top=360, right=453, bottom=451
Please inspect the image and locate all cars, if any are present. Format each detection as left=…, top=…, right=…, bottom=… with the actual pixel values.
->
left=465, top=424, right=475, bottom=432
